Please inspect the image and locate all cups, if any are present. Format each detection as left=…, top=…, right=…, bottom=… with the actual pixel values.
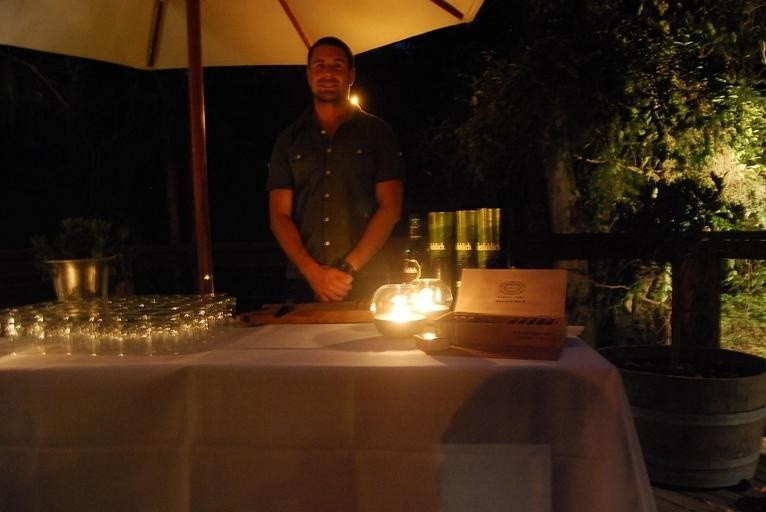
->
left=2, top=295, right=240, bottom=357
left=430, top=208, right=501, bottom=251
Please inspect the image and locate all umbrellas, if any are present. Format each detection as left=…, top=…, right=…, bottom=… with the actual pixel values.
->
left=0, top=1, right=483, bottom=295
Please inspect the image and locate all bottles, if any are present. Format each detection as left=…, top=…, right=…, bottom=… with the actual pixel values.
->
left=400, top=218, right=429, bottom=283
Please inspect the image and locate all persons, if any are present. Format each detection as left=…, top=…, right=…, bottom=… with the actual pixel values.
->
left=262, top=34, right=404, bottom=304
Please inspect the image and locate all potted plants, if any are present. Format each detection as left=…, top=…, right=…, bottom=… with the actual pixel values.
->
left=558, top=0, right=763, bottom=493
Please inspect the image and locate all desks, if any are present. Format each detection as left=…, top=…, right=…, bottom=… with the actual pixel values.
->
left=1, top=323, right=619, bottom=512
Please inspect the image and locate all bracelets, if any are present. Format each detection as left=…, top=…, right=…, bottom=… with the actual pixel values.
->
left=338, top=260, right=359, bottom=277
